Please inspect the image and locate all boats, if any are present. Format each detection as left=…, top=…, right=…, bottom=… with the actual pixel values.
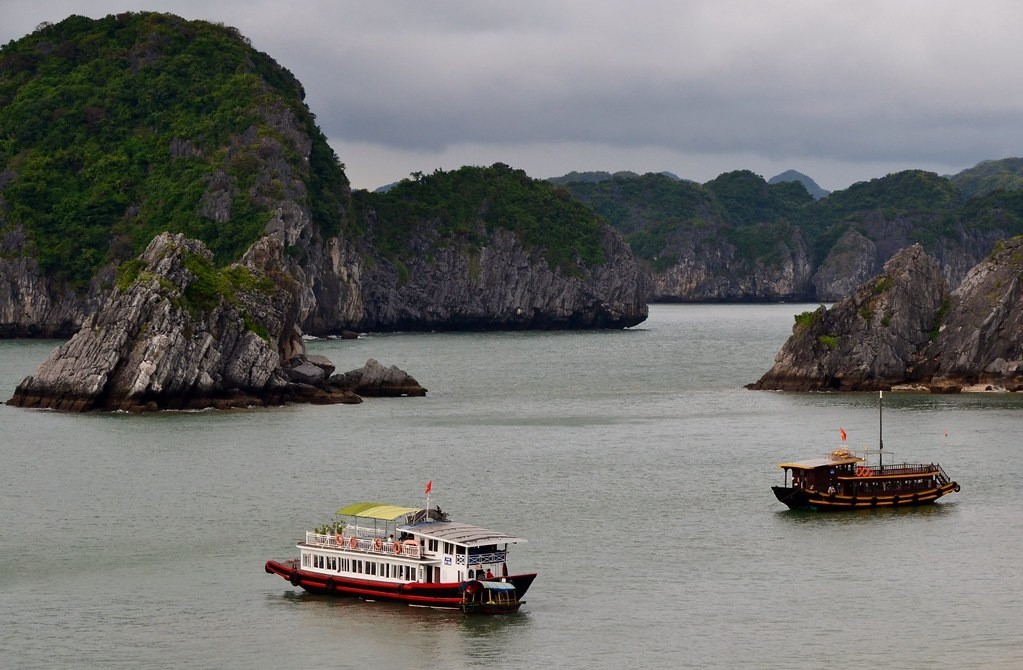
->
left=263, top=506, right=537, bottom=617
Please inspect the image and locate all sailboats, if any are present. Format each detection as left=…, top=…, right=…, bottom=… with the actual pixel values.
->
left=770, top=389, right=960, bottom=514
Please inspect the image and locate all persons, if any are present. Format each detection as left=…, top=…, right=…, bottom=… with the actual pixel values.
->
left=828, top=484, right=836, bottom=494
left=478, top=572, right=485, bottom=579
left=487, top=569, right=494, bottom=578
left=401, top=532, right=407, bottom=540
left=388, top=534, right=394, bottom=542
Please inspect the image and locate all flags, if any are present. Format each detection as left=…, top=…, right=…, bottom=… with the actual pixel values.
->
left=425, top=481, right=431, bottom=493
left=840, top=427, right=847, bottom=441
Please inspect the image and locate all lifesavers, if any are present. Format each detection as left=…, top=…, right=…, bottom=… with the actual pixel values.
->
left=375, top=537, right=382, bottom=549
left=954, top=484, right=960, bottom=492
left=867, top=468, right=873, bottom=476
left=350, top=537, right=357, bottom=548
left=335, top=534, right=343, bottom=545
left=862, top=467, right=868, bottom=476
left=912, top=494, right=919, bottom=501
left=870, top=497, right=878, bottom=505
left=828, top=494, right=836, bottom=502
left=937, top=488, right=943, bottom=496
left=290, top=572, right=300, bottom=587
left=892, top=496, right=900, bottom=504
left=393, top=542, right=401, bottom=553
left=326, top=578, right=336, bottom=593
left=850, top=497, right=857, bottom=505
left=856, top=468, right=862, bottom=476
